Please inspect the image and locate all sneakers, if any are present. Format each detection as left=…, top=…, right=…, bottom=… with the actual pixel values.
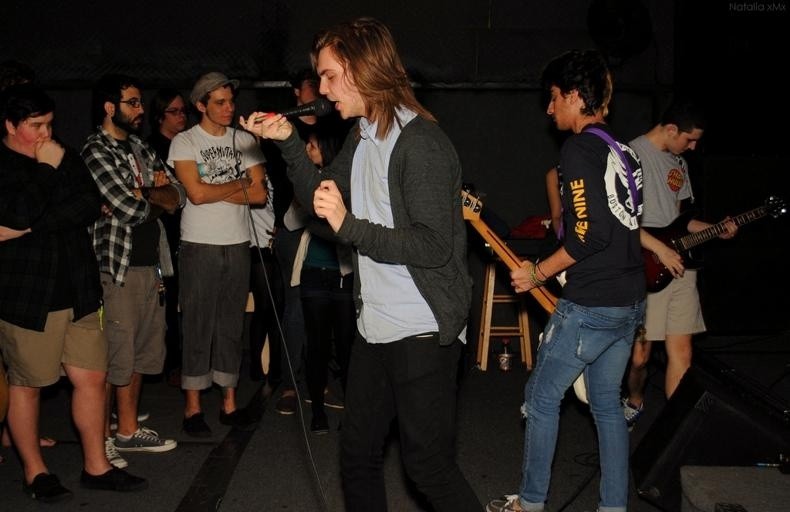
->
left=23, top=472, right=72, bottom=502
left=310, top=409, right=331, bottom=435
left=79, top=464, right=150, bottom=492
left=115, top=430, right=179, bottom=453
left=184, top=411, right=214, bottom=439
left=621, top=397, right=646, bottom=433
left=277, top=395, right=299, bottom=415
left=105, top=439, right=130, bottom=467
left=218, top=407, right=259, bottom=432
left=484, top=494, right=522, bottom=512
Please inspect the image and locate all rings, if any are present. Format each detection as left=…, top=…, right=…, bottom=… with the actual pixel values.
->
left=730, top=234, right=735, bottom=237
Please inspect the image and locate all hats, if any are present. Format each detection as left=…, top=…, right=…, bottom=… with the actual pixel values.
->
left=188, top=72, right=241, bottom=105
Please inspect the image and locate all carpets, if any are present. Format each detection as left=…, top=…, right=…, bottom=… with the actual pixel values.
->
left=215, top=350, right=788, bottom=509
left=0, top=352, right=263, bottom=509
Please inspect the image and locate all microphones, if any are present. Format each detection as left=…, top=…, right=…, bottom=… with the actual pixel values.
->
left=254, top=97, right=331, bottom=122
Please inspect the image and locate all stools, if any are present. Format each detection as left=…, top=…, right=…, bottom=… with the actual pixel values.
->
left=472, top=252, right=536, bottom=373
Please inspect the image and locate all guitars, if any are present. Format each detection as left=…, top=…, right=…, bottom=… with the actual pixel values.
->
left=642, top=197, right=787, bottom=292
left=460, top=189, right=589, bottom=404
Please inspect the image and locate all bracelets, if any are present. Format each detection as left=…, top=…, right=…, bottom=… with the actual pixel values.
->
left=530, top=264, right=548, bottom=287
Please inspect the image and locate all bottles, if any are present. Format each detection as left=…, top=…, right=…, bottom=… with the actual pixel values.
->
left=496, top=337, right=514, bottom=374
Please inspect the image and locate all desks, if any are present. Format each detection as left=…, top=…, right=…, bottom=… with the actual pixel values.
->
left=463, top=233, right=563, bottom=258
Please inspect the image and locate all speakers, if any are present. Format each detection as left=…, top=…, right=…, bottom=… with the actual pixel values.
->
left=631, top=361, right=790, bottom=512
left=680, top=465, right=790, bottom=512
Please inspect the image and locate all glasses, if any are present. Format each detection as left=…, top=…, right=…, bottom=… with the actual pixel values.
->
left=120, top=99, right=146, bottom=109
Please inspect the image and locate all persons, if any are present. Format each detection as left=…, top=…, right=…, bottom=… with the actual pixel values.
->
left=546, top=167, right=564, bottom=240
left=485, top=47, right=647, bottom=512
left=1, top=56, right=356, bottom=504
left=621, top=102, right=739, bottom=432
left=239, top=17, right=485, bottom=512
